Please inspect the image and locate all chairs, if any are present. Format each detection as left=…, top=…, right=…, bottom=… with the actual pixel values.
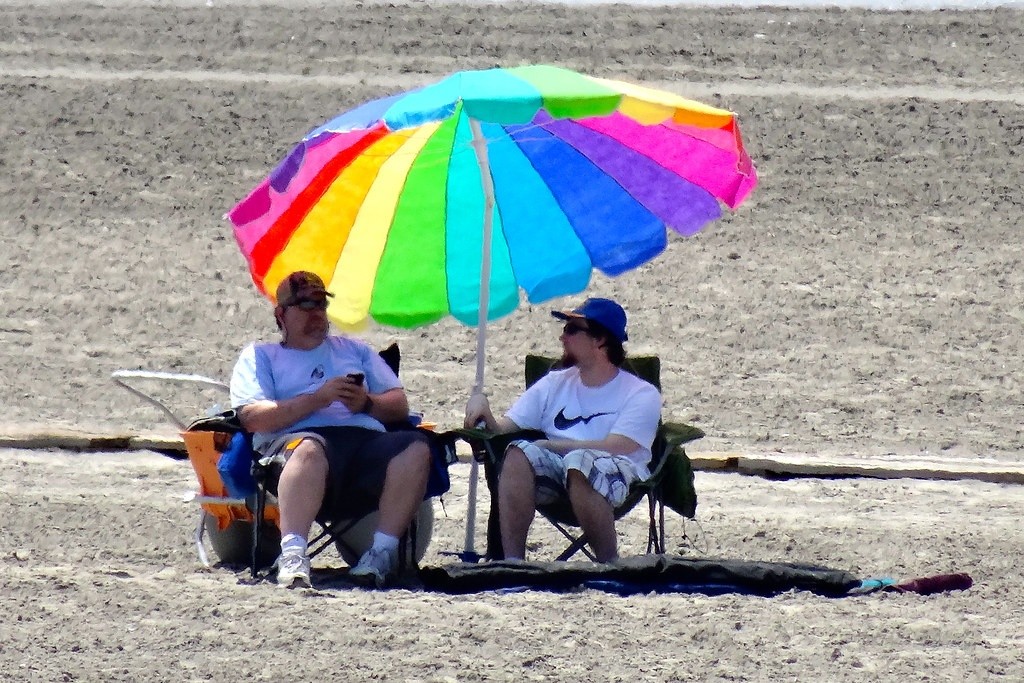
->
left=447, top=356, right=705, bottom=563
left=209, top=343, right=450, bottom=580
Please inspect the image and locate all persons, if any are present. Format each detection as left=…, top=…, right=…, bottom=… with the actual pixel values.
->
left=464, top=298, right=661, bottom=560
left=229, top=271, right=432, bottom=588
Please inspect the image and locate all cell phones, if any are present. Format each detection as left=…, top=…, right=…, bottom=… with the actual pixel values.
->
left=346, top=373, right=365, bottom=386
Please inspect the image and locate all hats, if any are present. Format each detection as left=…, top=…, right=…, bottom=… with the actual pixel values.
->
left=552, top=297, right=628, bottom=342
left=277, top=271, right=334, bottom=306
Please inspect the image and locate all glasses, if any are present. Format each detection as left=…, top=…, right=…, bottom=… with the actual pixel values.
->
left=564, top=323, right=599, bottom=336
left=285, top=299, right=328, bottom=311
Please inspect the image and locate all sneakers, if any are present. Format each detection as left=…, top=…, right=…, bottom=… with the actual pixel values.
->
left=349, top=547, right=388, bottom=589
left=277, top=547, right=311, bottom=588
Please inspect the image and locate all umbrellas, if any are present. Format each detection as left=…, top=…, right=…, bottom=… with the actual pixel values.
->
left=229, top=65, right=757, bottom=556
